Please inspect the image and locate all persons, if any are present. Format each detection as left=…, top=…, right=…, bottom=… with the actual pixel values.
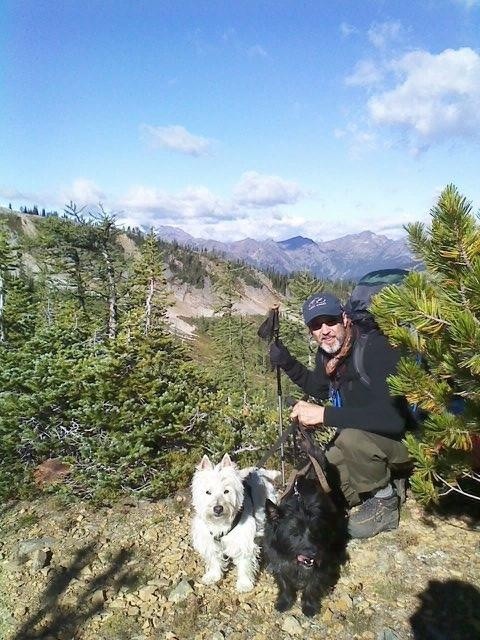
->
left=407, top=576, right=480, bottom=640
left=269, top=292, right=413, bottom=540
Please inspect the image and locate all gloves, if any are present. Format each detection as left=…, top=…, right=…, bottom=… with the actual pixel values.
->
left=269, top=339, right=295, bottom=373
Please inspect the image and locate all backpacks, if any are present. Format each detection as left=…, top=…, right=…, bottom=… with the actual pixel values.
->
left=348, top=268, right=471, bottom=430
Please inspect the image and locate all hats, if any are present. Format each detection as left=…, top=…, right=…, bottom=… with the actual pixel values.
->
left=302, top=292, right=343, bottom=325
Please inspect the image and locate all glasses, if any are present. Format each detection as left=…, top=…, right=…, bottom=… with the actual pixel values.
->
left=308, top=318, right=343, bottom=331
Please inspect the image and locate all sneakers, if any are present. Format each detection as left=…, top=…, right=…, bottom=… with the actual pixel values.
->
left=346, top=491, right=400, bottom=539
left=389, top=471, right=409, bottom=504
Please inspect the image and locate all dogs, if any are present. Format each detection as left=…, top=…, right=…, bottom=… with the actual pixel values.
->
left=187, top=452, right=282, bottom=595
left=263, top=463, right=353, bottom=618
